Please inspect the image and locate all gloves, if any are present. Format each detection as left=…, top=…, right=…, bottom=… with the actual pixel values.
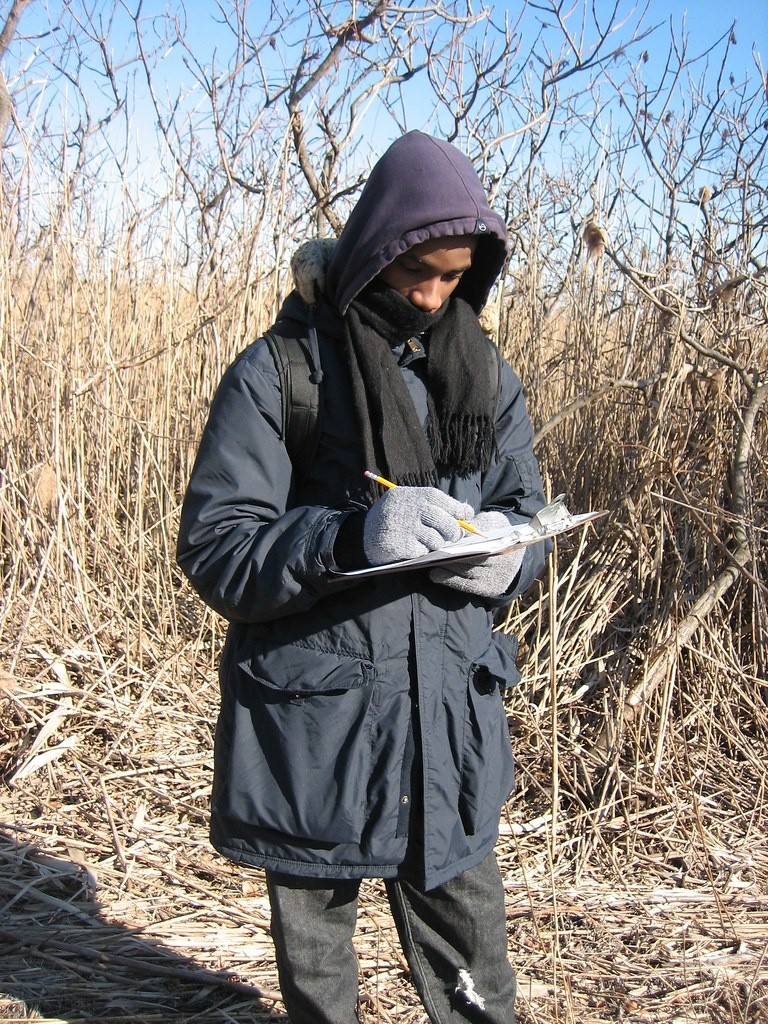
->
left=429, top=509, right=527, bottom=599
left=363, top=486, right=474, bottom=566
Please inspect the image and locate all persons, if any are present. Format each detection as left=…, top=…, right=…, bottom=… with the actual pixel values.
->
left=175, top=128, right=553, bottom=1024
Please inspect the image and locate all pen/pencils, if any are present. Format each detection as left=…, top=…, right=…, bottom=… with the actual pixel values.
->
left=364, top=470, right=489, bottom=540
